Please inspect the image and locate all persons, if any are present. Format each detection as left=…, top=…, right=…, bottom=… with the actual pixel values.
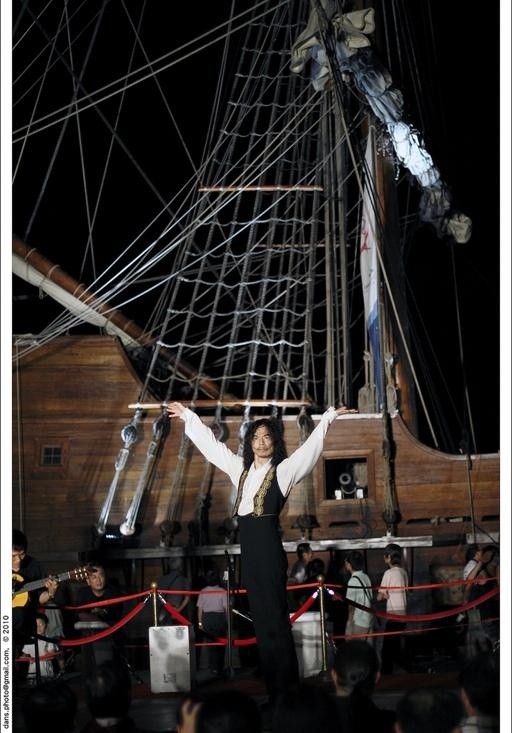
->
left=11, top=529, right=500, bottom=733
left=166, top=399, right=363, bottom=710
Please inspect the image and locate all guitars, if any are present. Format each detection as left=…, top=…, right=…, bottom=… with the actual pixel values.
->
left=12, top=563, right=98, bottom=608
